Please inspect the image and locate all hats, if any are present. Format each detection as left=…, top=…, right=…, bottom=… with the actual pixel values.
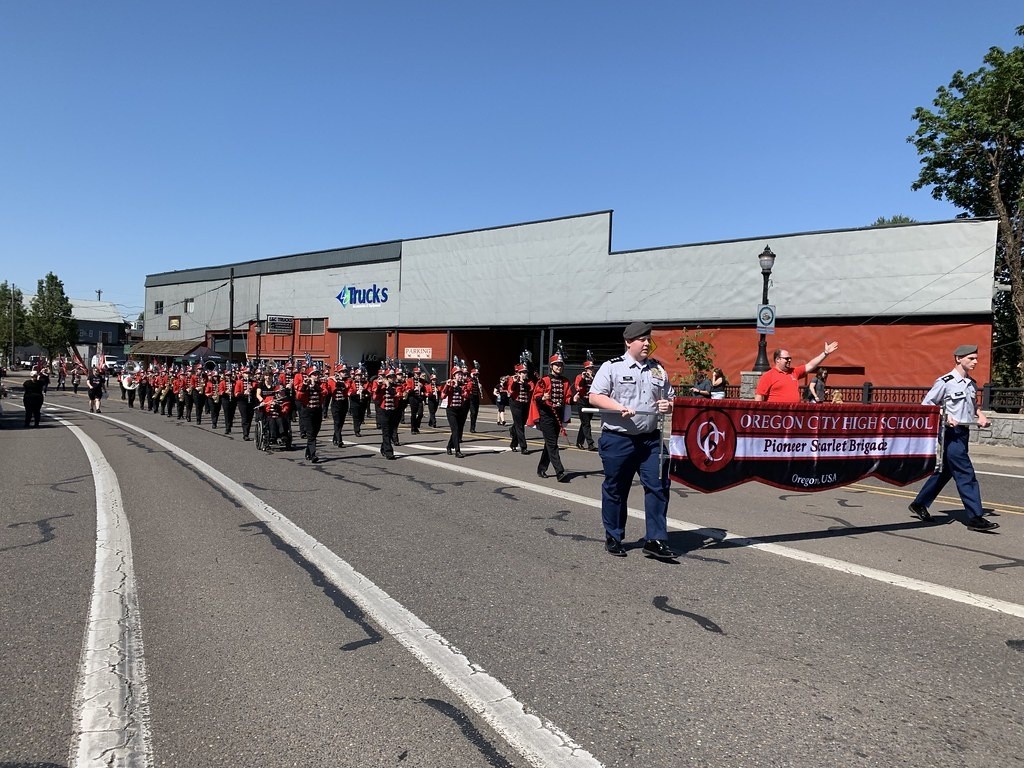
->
left=623, top=322, right=652, bottom=340
left=953, top=344, right=977, bottom=356
left=584, top=361, right=595, bottom=369
left=517, top=363, right=529, bottom=373
left=514, top=364, right=519, bottom=371
left=35, top=361, right=479, bottom=394
left=550, top=355, right=564, bottom=365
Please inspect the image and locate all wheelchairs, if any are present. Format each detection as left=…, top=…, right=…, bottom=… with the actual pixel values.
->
left=253, top=406, right=294, bottom=453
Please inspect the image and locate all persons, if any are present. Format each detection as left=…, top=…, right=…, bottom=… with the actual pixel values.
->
left=711, top=367, right=727, bottom=400
left=23, top=371, right=45, bottom=428
left=573, top=361, right=599, bottom=451
left=689, top=370, right=712, bottom=398
left=37, top=359, right=540, bottom=464
left=755, top=340, right=839, bottom=402
left=526, top=355, right=574, bottom=482
left=808, top=368, right=829, bottom=403
left=908, top=345, right=1000, bottom=530
left=589, top=319, right=681, bottom=559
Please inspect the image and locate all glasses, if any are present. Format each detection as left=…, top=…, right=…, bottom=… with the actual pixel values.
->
left=778, top=356, right=792, bottom=361
left=822, top=372, right=827, bottom=375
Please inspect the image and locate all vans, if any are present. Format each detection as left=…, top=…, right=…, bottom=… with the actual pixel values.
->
left=91, top=355, right=118, bottom=372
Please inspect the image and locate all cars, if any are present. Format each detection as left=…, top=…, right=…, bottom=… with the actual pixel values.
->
left=30, top=364, right=44, bottom=378
left=64, top=357, right=75, bottom=369
left=114, top=360, right=126, bottom=374
left=123, top=359, right=144, bottom=374
left=50, top=357, right=64, bottom=369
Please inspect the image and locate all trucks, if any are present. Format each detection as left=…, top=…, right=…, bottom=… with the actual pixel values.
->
left=20, top=356, right=47, bottom=369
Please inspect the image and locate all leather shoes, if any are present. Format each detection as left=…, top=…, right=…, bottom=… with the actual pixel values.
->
left=119, top=396, right=477, bottom=462
left=576, top=442, right=585, bottom=450
left=641, top=540, right=678, bottom=559
left=537, top=471, right=548, bottom=477
left=908, top=502, right=936, bottom=523
left=604, top=538, right=626, bottom=555
left=555, top=472, right=572, bottom=481
left=510, top=444, right=517, bottom=453
left=520, top=448, right=529, bottom=454
left=588, top=444, right=598, bottom=452
left=967, top=518, right=1000, bottom=532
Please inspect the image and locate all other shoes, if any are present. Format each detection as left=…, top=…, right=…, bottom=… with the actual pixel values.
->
left=502, top=421, right=506, bottom=425
left=497, top=421, right=501, bottom=425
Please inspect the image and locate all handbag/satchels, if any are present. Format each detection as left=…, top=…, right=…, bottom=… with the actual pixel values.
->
left=802, top=377, right=819, bottom=400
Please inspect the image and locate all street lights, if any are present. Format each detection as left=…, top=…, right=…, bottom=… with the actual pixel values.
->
left=751, top=243, right=778, bottom=373
